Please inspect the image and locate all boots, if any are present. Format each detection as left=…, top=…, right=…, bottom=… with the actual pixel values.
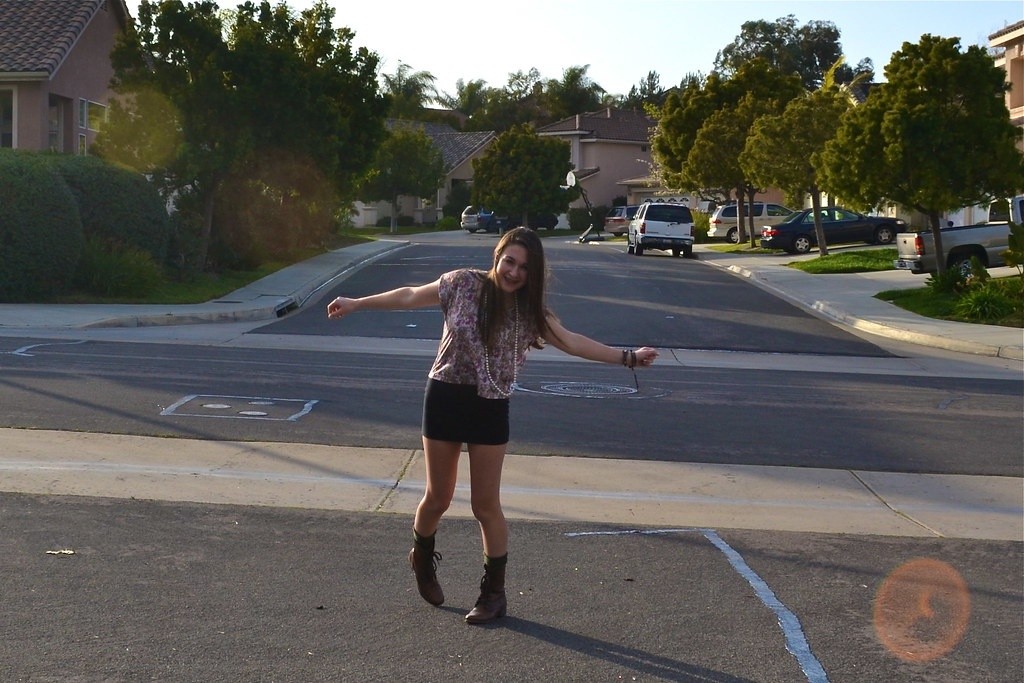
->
left=465, top=551, right=508, bottom=623
left=409, top=522, right=444, bottom=605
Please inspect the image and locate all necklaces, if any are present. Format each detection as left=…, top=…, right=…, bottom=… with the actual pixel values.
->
left=483, top=292, right=519, bottom=396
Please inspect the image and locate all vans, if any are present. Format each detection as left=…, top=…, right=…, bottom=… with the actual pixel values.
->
left=604, top=205, right=641, bottom=237
left=708, top=202, right=813, bottom=243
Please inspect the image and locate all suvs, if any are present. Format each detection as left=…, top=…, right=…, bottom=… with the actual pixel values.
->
left=460, top=205, right=493, bottom=233
left=628, top=202, right=695, bottom=258
left=488, top=208, right=558, bottom=231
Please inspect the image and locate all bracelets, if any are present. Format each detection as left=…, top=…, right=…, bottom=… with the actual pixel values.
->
left=630, top=350, right=639, bottom=389
left=623, top=349, right=628, bottom=366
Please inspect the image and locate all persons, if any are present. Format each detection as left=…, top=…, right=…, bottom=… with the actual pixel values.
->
left=326, top=227, right=659, bottom=625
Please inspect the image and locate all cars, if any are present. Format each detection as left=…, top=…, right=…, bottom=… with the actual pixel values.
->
left=781, top=207, right=908, bottom=254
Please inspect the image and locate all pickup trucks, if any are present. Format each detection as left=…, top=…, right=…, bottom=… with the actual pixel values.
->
left=892, top=193, right=1024, bottom=284
